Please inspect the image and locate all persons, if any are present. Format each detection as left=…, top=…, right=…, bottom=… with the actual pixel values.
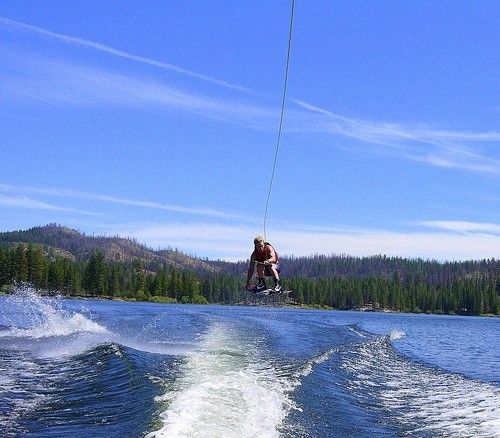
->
left=245, top=237, right=282, bottom=294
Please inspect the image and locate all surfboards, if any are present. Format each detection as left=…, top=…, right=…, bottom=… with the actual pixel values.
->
left=244, top=283, right=292, bottom=298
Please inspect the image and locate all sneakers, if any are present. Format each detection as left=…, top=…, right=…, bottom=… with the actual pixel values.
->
left=269, top=278, right=283, bottom=294
left=256, top=279, right=267, bottom=291
